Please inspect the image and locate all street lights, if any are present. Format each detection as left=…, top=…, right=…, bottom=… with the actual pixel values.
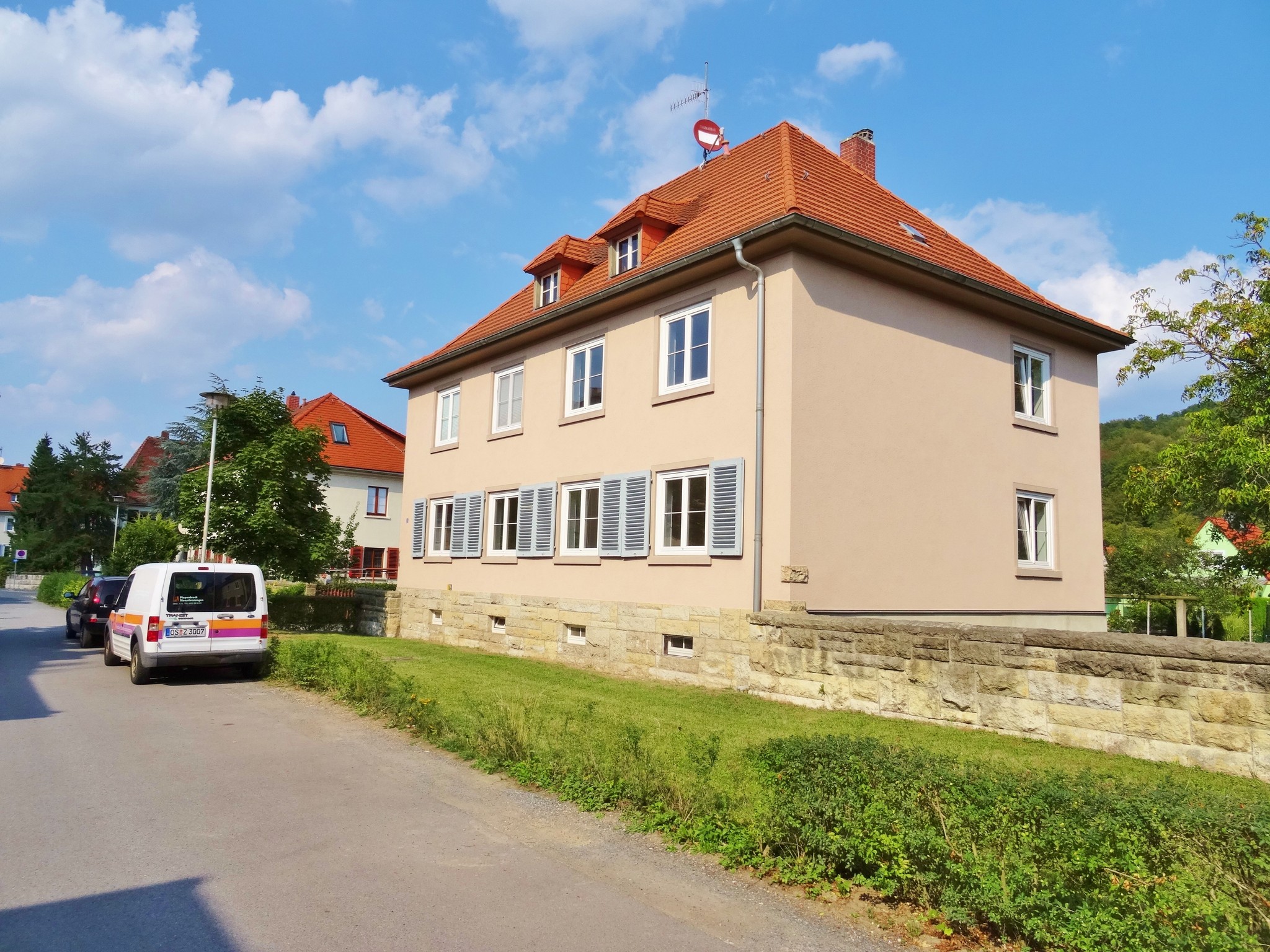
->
left=198, top=392, right=238, bottom=563
left=109, top=495, right=126, bottom=567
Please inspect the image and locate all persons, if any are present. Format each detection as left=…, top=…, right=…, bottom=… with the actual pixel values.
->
left=320, top=572, right=332, bottom=586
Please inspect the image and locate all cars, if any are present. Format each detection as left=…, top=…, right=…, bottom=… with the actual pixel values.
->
left=62, top=576, right=129, bottom=650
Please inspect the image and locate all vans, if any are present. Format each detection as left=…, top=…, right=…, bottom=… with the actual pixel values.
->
left=103, top=561, right=270, bottom=685
